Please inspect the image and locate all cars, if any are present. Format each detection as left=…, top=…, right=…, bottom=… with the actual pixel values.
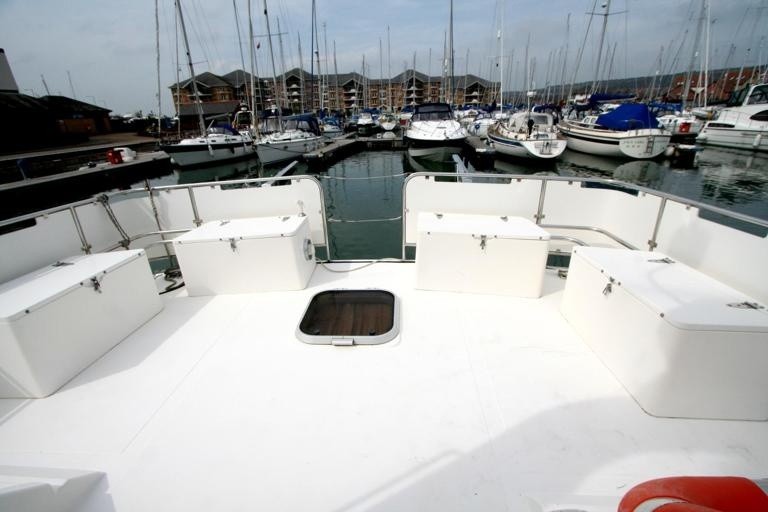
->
left=108, top=146, right=138, bottom=162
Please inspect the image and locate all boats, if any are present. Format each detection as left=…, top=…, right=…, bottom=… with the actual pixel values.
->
left=0, top=167, right=768, bottom=512
